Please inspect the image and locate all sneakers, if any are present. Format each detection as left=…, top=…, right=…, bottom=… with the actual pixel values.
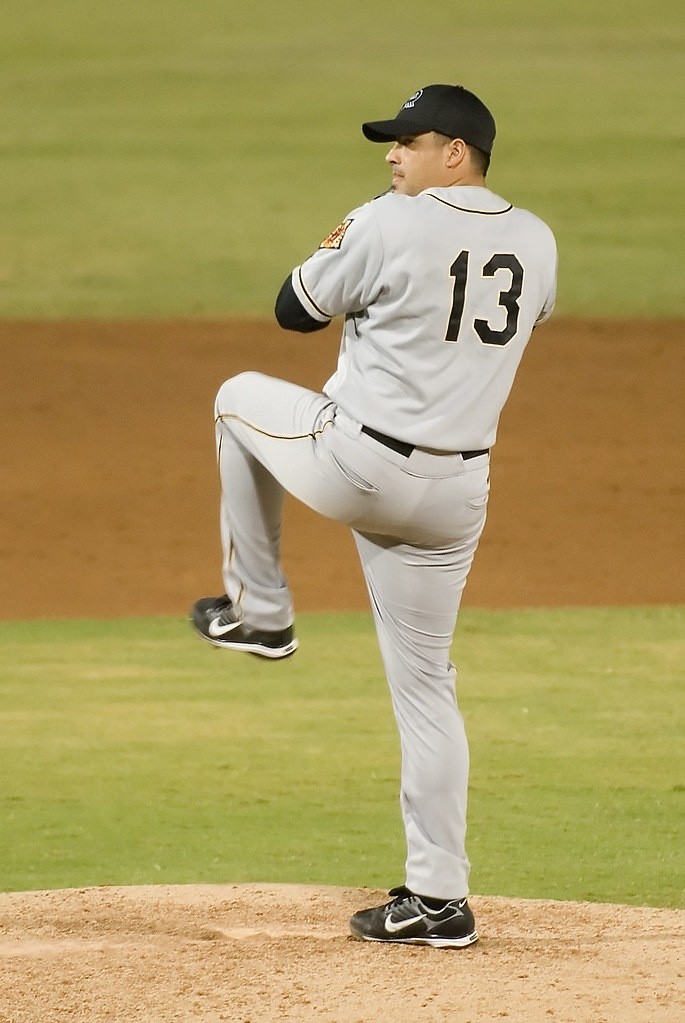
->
left=190, top=593, right=298, bottom=658
left=349, top=885, right=478, bottom=947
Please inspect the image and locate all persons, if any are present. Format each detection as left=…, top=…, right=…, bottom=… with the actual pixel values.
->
left=191, top=84, right=559, bottom=947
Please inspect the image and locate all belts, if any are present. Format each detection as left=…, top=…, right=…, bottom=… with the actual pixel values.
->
left=362, top=426, right=489, bottom=460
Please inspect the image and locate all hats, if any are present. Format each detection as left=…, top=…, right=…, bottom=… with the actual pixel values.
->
left=362, top=83, right=497, bottom=154
